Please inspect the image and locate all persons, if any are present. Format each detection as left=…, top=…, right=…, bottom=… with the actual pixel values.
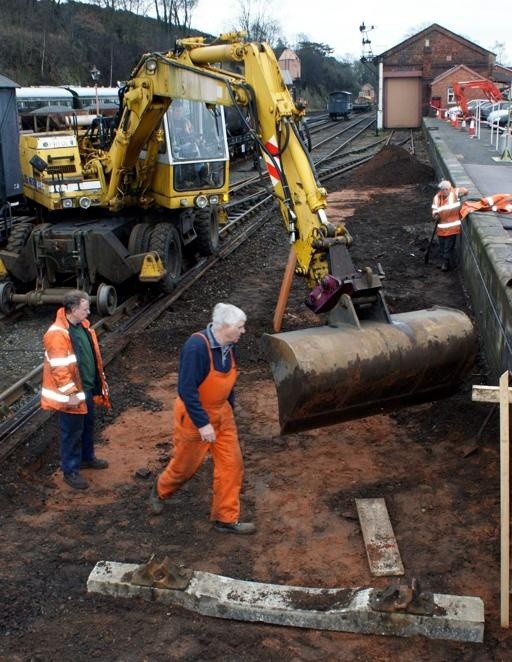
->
left=170, top=101, right=209, bottom=180
left=39, top=287, right=112, bottom=489
left=147, top=301, right=257, bottom=536
left=431, top=179, right=469, bottom=273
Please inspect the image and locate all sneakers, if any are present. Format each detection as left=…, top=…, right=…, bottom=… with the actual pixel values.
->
left=436, top=255, right=442, bottom=269
left=442, top=259, right=449, bottom=271
left=83, top=457, right=108, bottom=468
left=149, top=476, right=165, bottom=515
left=63, top=472, right=90, bottom=490
left=214, top=521, right=256, bottom=534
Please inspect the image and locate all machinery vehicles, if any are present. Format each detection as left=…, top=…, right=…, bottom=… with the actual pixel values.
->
left=451, top=80, right=512, bottom=120
left=2, top=34, right=479, bottom=436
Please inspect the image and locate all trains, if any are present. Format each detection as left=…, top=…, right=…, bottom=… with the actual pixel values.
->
left=328, top=91, right=376, bottom=121
left=16, top=82, right=120, bottom=127
left=1, top=75, right=299, bottom=216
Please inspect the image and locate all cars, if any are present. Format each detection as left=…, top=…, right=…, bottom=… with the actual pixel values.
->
left=445, top=98, right=512, bottom=133
